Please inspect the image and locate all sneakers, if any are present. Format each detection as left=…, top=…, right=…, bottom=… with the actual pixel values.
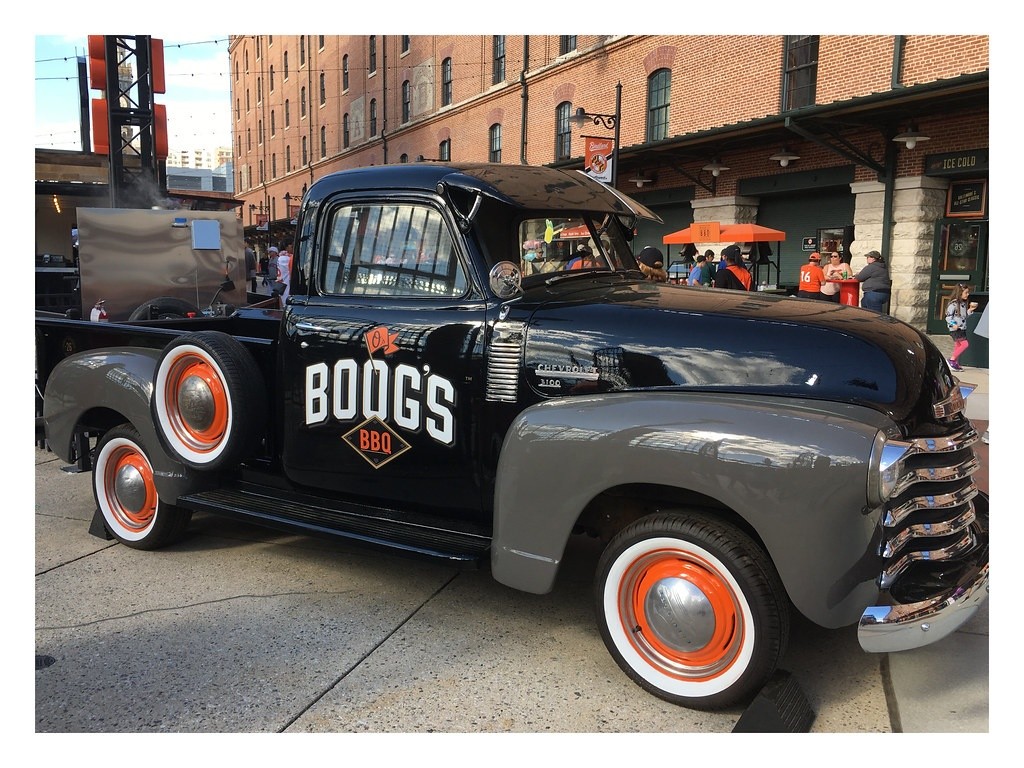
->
left=946, top=358, right=957, bottom=370
left=954, top=364, right=964, bottom=372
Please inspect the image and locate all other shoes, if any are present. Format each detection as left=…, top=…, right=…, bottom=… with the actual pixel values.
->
left=262, top=283, right=265, bottom=287
left=265, top=282, right=268, bottom=286
left=981, top=431, right=989, bottom=444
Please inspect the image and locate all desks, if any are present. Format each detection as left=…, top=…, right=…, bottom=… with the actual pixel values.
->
left=826, top=280, right=859, bottom=306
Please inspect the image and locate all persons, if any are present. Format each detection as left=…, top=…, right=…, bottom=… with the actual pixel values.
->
left=981, top=426, right=989, bottom=445
left=944, top=282, right=978, bottom=372
left=244, top=237, right=256, bottom=292
left=563, top=240, right=617, bottom=271
left=532, top=247, right=544, bottom=263
left=637, top=248, right=668, bottom=283
left=797, top=252, right=826, bottom=299
left=688, top=250, right=716, bottom=288
left=267, top=243, right=294, bottom=308
left=716, top=245, right=753, bottom=291
left=821, top=250, right=852, bottom=304
left=854, top=251, right=891, bottom=313
left=259, top=253, right=270, bottom=287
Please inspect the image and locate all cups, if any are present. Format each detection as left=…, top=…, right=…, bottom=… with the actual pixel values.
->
left=843, top=271, right=847, bottom=280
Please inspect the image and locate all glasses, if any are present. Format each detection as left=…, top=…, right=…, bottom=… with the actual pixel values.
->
left=830, top=256, right=839, bottom=258
left=538, top=251, right=543, bottom=254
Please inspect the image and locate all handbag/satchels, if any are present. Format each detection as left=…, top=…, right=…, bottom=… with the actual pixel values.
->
left=272, top=282, right=287, bottom=296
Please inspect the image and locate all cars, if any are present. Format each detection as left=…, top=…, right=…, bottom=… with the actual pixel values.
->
left=35, top=156, right=990, bottom=714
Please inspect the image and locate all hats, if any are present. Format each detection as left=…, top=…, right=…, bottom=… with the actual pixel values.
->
left=577, top=244, right=584, bottom=251
left=580, top=246, right=593, bottom=254
left=809, top=252, right=821, bottom=260
left=637, top=247, right=664, bottom=269
left=864, top=251, right=880, bottom=259
left=602, top=240, right=610, bottom=249
left=244, top=236, right=252, bottom=245
left=266, top=247, right=278, bottom=255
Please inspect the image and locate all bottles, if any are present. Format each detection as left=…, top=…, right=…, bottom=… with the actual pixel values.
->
left=761, top=280, right=767, bottom=286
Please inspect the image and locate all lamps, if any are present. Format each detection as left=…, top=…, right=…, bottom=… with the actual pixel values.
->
left=628, top=159, right=652, bottom=188
left=892, top=114, right=931, bottom=150
left=701, top=150, right=731, bottom=177
left=769, top=139, right=800, bottom=167
